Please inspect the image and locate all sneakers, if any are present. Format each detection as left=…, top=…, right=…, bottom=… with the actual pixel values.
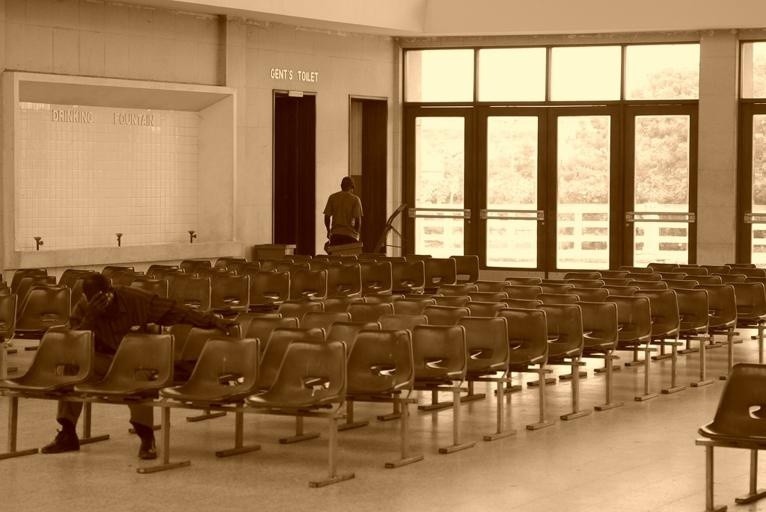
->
left=42, top=430, right=80, bottom=453
left=137, top=436, right=157, bottom=459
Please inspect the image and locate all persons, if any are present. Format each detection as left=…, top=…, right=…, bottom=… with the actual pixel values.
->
left=323, top=176, right=364, bottom=245
